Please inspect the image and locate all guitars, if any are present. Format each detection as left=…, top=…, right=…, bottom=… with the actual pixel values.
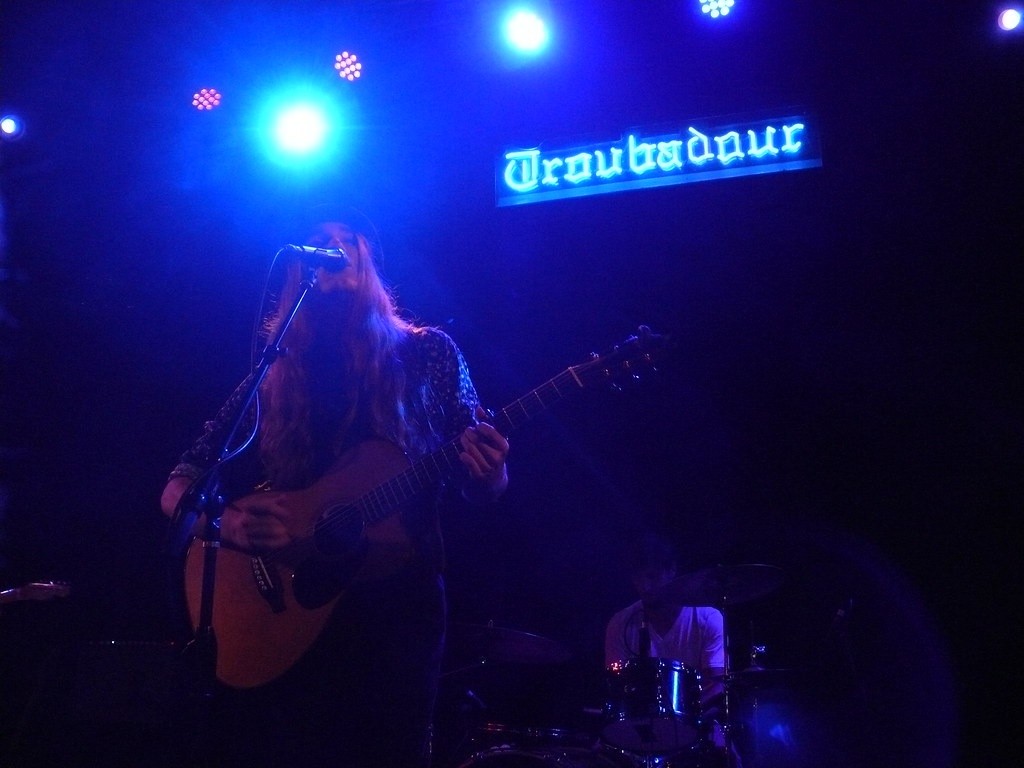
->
left=181, top=325, right=679, bottom=693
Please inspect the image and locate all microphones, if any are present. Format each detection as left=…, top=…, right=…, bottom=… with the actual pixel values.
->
left=284, top=244, right=346, bottom=273
left=639, top=603, right=651, bottom=655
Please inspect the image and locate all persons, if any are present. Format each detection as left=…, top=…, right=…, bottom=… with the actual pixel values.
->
left=161, top=202, right=509, bottom=768
left=603, top=566, right=725, bottom=721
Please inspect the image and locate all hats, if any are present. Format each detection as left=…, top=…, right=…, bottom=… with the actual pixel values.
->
left=285, top=202, right=385, bottom=278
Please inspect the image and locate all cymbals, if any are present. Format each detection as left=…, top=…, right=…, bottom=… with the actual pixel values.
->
left=739, top=664, right=769, bottom=673
left=446, top=621, right=560, bottom=667
left=658, top=564, right=783, bottom=605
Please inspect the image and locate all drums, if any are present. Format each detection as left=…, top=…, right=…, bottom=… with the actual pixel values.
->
left=479, top=721, right=595, bottom=749
left=459, top=747, right=619, bottom=768
left=600, top=656, right=706, bottom=757
